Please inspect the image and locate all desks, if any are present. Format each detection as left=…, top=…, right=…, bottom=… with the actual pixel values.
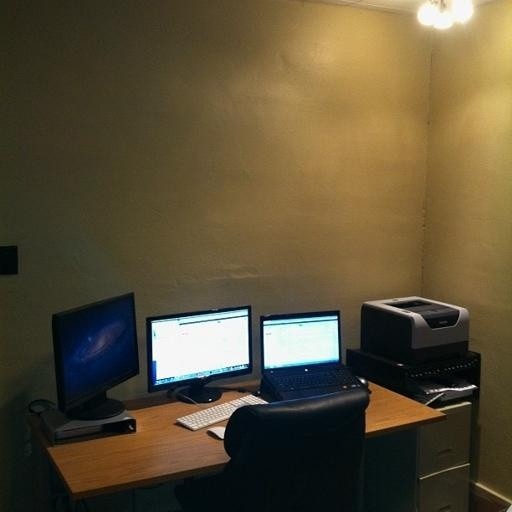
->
left=25, top=380, right=451, bottom=512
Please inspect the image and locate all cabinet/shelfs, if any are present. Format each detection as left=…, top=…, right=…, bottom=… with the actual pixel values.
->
left=346, top=348, right=481, bottom=512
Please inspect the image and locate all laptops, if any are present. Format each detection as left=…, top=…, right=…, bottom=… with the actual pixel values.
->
left=259, top=310, right=372, bottom=402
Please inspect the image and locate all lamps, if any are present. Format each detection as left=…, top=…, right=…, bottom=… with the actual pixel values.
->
left=417, top=0, right=473, bottom=30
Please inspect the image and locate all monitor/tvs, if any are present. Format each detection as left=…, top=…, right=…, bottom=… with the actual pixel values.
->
left=146, top=305, right=253, bottom=404
left=51, top=291, right=140, bottom=421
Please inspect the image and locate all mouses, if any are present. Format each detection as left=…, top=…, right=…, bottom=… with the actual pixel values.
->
left=207, top=426, right=227, bottom=440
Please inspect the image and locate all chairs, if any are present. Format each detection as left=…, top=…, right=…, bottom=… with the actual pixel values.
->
left=223, top=388, right=370, bottom=512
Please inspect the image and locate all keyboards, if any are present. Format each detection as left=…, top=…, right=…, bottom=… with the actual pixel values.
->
left=176, top=394, right=270, bottom=432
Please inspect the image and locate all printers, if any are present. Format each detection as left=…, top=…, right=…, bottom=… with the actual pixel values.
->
left=360, top=296, right=470, bottom=368
